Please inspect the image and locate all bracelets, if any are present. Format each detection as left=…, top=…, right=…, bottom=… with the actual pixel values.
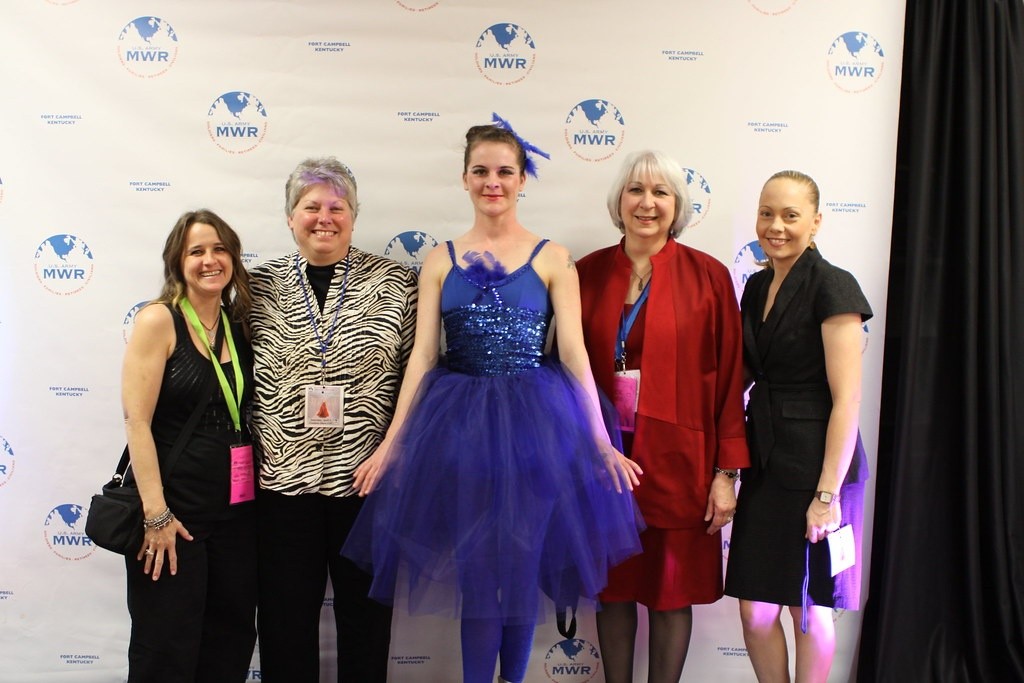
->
left=714, top=466, right=741, bottom=482
left=143, top=508, right=173, bottom=530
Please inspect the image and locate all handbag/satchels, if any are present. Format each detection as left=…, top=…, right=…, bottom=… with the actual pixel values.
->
left=85, top=477, right=145, bottom=556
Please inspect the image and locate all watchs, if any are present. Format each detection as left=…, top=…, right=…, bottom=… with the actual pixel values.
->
left=812, top=488, right=840, bottom=507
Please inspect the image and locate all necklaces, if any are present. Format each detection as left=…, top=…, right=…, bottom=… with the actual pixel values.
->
left=197, top=310, right=221, bottom=352
left=631, top=267, right=651, bottom=290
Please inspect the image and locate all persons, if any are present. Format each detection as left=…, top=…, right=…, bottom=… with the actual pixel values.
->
left=233, top=159, right=421, bottom=683
left=120, top=209, right=257, bottom=683
left=554, top=147, right=750, bottom=683
left=725, top=168, right=879, bottom=683
left=351, top=124, right=644, bottom=683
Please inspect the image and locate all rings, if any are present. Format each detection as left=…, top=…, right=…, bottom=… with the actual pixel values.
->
left=145, top=549, right=154, bottom=558
